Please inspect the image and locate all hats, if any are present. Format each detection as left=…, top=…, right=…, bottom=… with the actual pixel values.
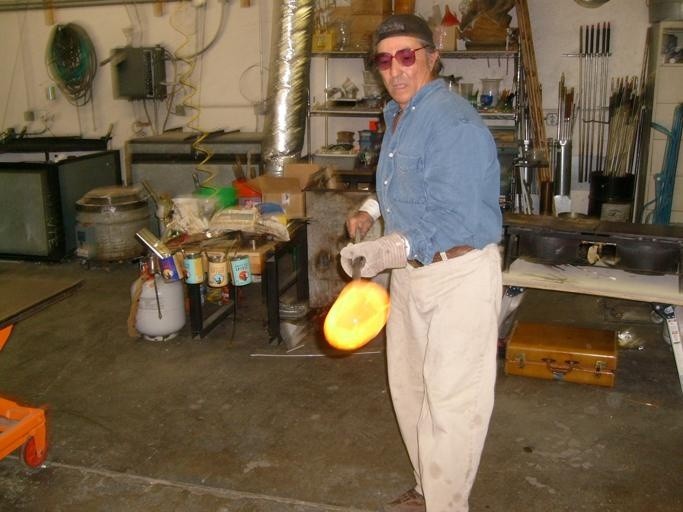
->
left=373, top=14, right=433, bottom=47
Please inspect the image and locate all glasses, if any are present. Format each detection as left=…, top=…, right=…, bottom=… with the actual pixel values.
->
left=375, top=42, right=435, bottom=69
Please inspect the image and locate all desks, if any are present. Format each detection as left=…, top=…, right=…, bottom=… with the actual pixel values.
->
left=163, top=212, right=306, bottom=347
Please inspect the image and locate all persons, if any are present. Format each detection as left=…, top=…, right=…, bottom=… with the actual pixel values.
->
left=340, top=13, right=504, bottom=508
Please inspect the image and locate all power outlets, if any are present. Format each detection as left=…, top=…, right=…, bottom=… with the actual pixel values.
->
left=37, top=110, right=47, bottom=120
left=25, top=111, right=33, bottom=121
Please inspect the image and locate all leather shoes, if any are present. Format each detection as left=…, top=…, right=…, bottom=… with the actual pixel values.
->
left=384, top=489, right=425, bottom=511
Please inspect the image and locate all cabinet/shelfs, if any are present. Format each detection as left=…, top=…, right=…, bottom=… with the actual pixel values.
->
left=0, top=138, right=121, bottom=262
left=635, top=19, right=683, bottom=226
left=306, top=51, right=524, bottom=213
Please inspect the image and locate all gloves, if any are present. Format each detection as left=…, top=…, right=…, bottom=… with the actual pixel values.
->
left=340, top=232, right=409, bottom=278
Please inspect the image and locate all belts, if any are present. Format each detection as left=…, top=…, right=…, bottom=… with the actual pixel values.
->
left=405, top=242, right=475, bottom=267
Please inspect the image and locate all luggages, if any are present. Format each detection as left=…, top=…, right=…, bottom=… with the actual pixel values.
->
left=504, top=320, right=617, bottom=387
